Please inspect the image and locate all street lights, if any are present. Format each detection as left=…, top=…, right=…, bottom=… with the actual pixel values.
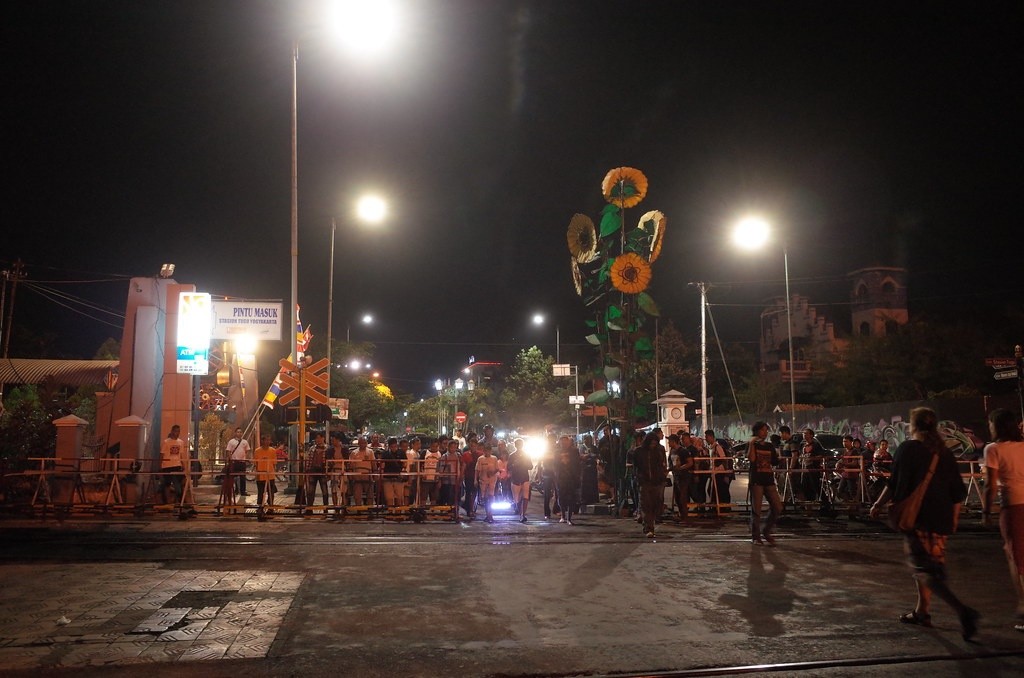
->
left=434, top=379, right=443, bottom=436
left=346, top=314, right=372, bottom=345
left=455, top=378, right=463, bottom=435
left=324, top=201, right=387, bottom=443
left=735, top=218, right=795, bottom=432
left=534, top=314, right=560, bottom=365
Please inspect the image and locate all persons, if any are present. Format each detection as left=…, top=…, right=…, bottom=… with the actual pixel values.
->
left=769, top=425, right=895, bottom=517
left=254, top=435, right=278, bottom=513
left=984, top=406, right=1024, bottom=631
left=869, top=408, right=981, bottom=639
left=226, top=428, right=251, bottom=496
left=743, top=421, right=783, bottom=545
left=160, top=425, right=186, bottom=504
left=305, top=425, right=735, bottom=536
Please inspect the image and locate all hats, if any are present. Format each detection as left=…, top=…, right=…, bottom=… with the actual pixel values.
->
left=665, top=434, right=679, bottom=439
left=386, top=436, right=397, bottom=442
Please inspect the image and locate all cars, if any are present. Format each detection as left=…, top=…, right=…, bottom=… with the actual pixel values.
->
left=813, top=433, right=847, bottom=458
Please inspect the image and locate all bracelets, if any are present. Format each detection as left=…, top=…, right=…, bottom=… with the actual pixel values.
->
left=873, top=502, right=881, bottom=508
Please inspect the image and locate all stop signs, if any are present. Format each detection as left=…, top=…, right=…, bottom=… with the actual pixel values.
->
left=456, top=412, right=467, bottom=424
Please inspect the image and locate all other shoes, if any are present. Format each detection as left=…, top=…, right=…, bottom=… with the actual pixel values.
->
left=753, top=538, right=764, bottom=545
left=677, top=519, right=687, bottom=523
left=646, top=532, right=654, bottom=538
left=762, top=531, right=777, bottom=546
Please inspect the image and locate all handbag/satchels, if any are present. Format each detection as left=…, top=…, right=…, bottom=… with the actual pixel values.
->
left=887, top=482, right=927, bottom=530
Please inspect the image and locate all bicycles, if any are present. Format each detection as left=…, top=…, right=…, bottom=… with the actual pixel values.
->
left=245, top=463, right=258, bottom=481
left=529, top=462, right=555, bottom=499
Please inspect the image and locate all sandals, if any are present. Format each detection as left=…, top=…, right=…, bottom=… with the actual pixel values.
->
left=960, top=608, right=980, bottom=641
left=900, top=609, right=930, bottom=626
